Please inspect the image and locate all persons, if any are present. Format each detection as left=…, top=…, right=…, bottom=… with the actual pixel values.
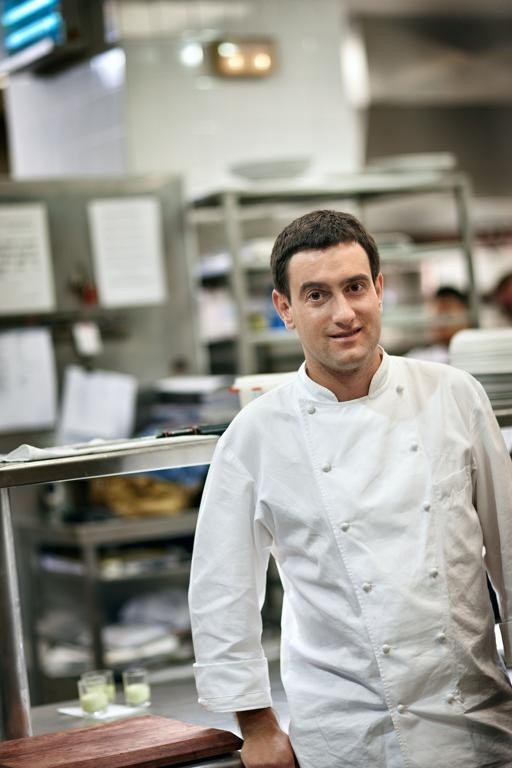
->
left=188, top=208, right=511, bottom=768
left=476, top=269, right=512, bottom=330
left=405, top=285, right=472, bottom=364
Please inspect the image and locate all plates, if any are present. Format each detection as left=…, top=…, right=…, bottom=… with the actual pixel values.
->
left=448, top=327, right=512, bottom=410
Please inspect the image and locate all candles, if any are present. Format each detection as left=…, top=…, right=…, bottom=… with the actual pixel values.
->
left=79, top=683, right=151, bottom=711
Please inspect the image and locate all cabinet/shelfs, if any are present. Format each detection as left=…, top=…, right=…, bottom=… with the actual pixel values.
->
left=11, top=508, right=199, bottom=683
left=191, top=165, right=479, bottom=379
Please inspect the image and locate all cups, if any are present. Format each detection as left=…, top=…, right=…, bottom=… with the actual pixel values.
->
left=77, top=669, right=116, bottom=718
left=121, top=666, right=152, bottom=710
